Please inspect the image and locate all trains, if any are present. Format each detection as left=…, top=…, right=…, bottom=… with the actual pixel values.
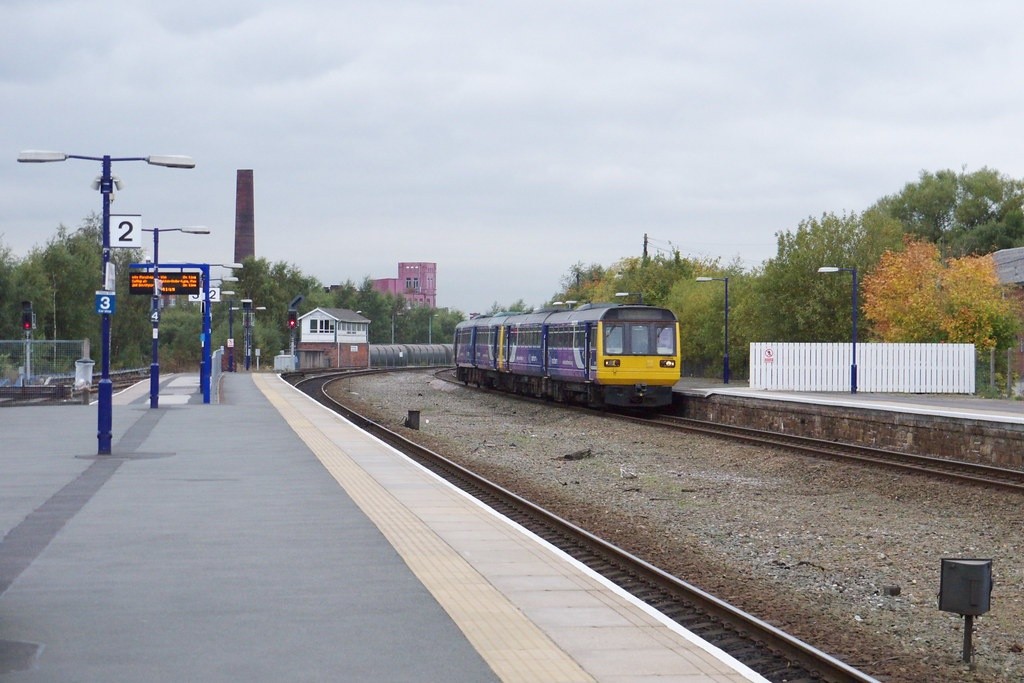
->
left=453, top=303, right=681, bottom=410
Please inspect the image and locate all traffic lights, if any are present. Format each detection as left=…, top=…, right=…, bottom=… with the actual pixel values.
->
left=289, top=311, right=299, bottom=329
left=22, top=301, right=33, bottom=330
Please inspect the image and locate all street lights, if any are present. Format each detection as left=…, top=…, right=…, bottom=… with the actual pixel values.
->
left=201, top=263, right=243, bottom=393
left=818, top=267, right=858, bottom=394
left=615, top=293, right=643, bottom=304
left=428, top=314, right=439, bottom=344
left=229, top=307, right=266, bottom=371
left=141, top=228, right=210, bottom=409
left=17, top=150, right=196, bottom=455
left=392, top=313, right=403, bottom=345
left=696, top=277, right=728, bottom=385
